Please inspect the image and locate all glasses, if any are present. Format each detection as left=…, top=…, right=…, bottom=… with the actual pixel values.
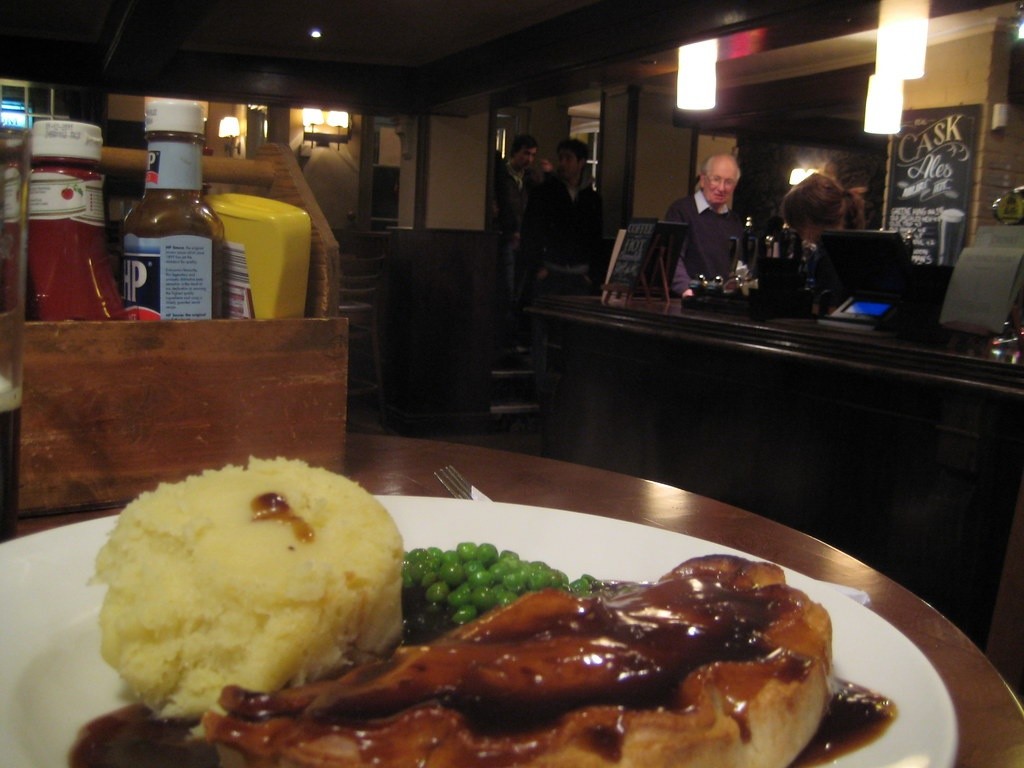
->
left=704, top=174, right=738, bottom=189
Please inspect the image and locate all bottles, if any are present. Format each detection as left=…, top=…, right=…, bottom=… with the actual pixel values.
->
left=119, top=97, right=224, bottom=321
left=25, top=120, right=126, bottom=321
left=204, top=192, right=313, bottom=320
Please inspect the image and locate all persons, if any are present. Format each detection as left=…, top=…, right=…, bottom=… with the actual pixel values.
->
left=491, top=134, right=606, bottom=352
left=784, top=173, right=864, bottom=250
left=659, top=151, right=752, bottom=296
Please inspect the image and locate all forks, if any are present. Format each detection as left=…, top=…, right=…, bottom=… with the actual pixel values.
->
left=434, top=463, right=481, bottom=499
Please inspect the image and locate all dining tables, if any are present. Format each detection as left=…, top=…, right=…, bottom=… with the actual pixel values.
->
left=0, top=429, right=1024, bottom=768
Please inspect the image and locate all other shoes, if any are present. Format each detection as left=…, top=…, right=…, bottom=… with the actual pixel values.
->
left=498, top=345, right=516, bottom=359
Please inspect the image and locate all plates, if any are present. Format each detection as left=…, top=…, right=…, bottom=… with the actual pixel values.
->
left=0, top=496, right=961, bottom=768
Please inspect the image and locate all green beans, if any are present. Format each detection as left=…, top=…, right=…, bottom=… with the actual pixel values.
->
left=403, top=538, right=607, bottom=626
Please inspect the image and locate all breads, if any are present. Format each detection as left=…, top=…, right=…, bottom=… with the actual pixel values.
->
left=200, top=553, right=836, bottom=768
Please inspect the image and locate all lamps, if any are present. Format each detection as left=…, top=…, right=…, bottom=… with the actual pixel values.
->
left=875, top=0, right=930, bottom=80
left=218, top=115, right=241, bottom=158
left=677, top=39, right=718, bottom=111
left=863, top=73, right=905, bottom=136
left=301, top=108, right=354, bottom=150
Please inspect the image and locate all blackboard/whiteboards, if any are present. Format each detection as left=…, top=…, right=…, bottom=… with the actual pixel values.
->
left=609, top=217, right=658, bottom=286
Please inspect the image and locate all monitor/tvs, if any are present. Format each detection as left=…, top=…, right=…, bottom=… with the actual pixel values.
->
left=820, top=230, right=924, bottom=300
left=0, top=97, right=36, bottom=130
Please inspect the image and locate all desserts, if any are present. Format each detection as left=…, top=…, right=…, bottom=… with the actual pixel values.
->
left=91, top=453, right=405, bottom=717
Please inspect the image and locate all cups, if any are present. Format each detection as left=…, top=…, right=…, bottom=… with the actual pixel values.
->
left=0, top=127, right=31, bottom=540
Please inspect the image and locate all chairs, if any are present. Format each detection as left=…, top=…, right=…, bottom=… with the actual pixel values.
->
left=340, top=253, right=388, bottom=427
